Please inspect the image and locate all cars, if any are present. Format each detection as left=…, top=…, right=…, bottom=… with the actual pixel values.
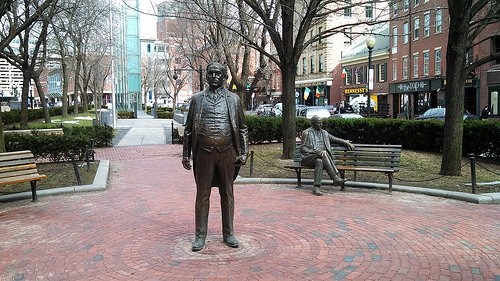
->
left=414, top=107, right=479, bottom=121
left=255, top=102, right=365, bottom=119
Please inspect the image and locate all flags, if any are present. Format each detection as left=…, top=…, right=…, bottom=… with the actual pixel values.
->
left=356, top=66, right=360, bottom=74
left=303, top=87, right=311, bottom=100
left=341, top=68, right=348, bottom=79
left=315, top=86, right=323, bottom=98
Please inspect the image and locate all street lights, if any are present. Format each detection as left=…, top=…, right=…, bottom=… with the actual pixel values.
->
left=172, top=64, right=203, bottom=91
left=365, top=36, right=376, bottom=118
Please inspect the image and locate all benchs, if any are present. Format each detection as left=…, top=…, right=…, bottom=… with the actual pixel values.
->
left=284, top=143, right=402, bottom=195
left=0, top=150, right=47, bottom=202
left=2, top=129, right=64, bottom=137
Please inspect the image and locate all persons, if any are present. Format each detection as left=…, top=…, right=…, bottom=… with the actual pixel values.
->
left=481, top=106, right=491, bottom=120
left=300, top=115, right=354, bottom=196
left=181, top=62, right=250, bottom=251
